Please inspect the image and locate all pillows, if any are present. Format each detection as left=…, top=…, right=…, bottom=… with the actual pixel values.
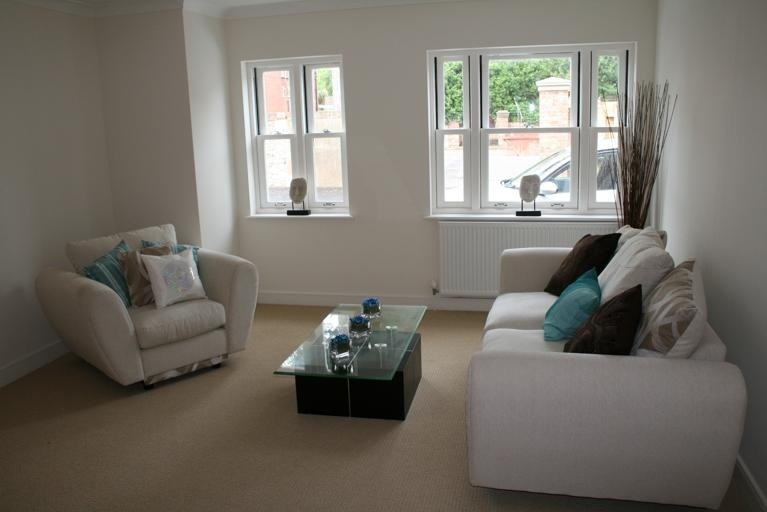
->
left=543, top=224, right=707, bottom=360
left=66, top=223, right=209, bottom=309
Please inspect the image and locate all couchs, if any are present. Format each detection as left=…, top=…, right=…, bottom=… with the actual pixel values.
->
left=465, top=247, right=748, bottom=509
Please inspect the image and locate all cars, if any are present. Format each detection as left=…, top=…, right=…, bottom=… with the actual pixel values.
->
left=488, top=144, right=619, bottom=206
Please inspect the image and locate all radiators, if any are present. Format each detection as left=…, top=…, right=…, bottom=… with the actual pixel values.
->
left=431, top=221, right=623, bottom=299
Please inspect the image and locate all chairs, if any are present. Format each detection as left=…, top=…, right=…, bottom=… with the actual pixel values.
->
left=37, top=224, right=259, bottom=391
